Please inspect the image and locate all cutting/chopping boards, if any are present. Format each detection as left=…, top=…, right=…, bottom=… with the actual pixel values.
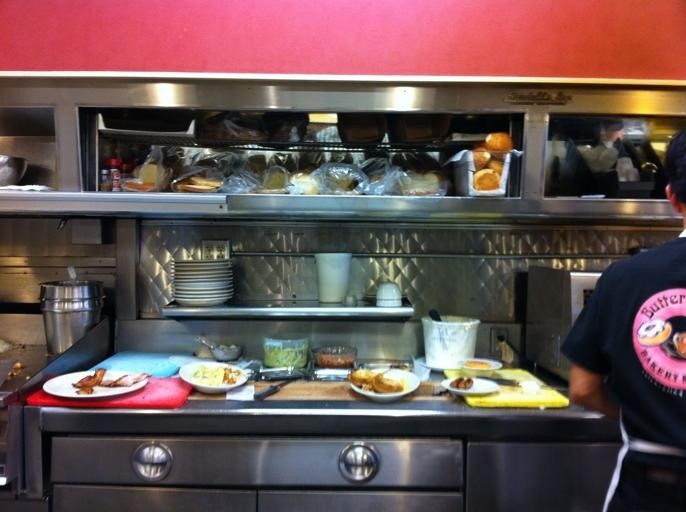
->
left=89, top=348, right=196, bottom=379
left=186, top=380, right=456, bottom=403
left=26, top=377, right=193, bottom=410
left=440, top=365, right=572, bottom=411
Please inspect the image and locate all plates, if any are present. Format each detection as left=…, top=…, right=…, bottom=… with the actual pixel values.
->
left=39, top=367, right=151, bottom=401
left=346, top=367, right=423, bottom=402
left=456, top=358, right=504, bottom=372
left=177, top=359, right=250, bottom=398
left=168, top=255, right=236, bottom=306
left=437, top=375, right=501, bottom=397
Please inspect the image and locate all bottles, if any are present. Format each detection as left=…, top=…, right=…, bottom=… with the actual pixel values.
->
left=100, top=156, right=130, bottom=192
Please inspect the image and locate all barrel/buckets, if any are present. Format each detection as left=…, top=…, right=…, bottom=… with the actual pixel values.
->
left=35, top=280, right=105, bottom=357
left=422, top=316, right=481, bottom=370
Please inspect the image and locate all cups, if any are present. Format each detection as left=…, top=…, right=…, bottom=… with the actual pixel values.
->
left=314, top=251, right=352, bottom=304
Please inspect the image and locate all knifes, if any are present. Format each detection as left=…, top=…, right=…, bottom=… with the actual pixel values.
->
left=254, top=379, right=298, bottom=401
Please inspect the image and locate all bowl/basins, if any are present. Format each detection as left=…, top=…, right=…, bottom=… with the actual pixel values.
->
left=311, top=342, right=359, bottom=370
left=374, top=281, right=403, bottom=308
left=209, top=343, right=243, bottom=362
left=0, top=155, right=28, bottom=186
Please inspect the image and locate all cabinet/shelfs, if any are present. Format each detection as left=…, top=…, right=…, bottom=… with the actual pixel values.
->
left=0, top=73, right=686, bottom=319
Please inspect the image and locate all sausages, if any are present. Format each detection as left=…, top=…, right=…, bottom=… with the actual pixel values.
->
left=451, top=377, right=474, bottom=389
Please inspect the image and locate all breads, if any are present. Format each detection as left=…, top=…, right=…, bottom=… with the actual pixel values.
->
left=468, top=132, right=513, bottom=191
left=173, top=176, right=223, bottom=192
left=125, top=163, right=166, bottom=193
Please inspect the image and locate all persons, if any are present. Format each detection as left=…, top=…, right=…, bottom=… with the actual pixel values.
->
left=601, top=118, right=640, bottom=174
left=558, top=127, right=686, bottom=510
